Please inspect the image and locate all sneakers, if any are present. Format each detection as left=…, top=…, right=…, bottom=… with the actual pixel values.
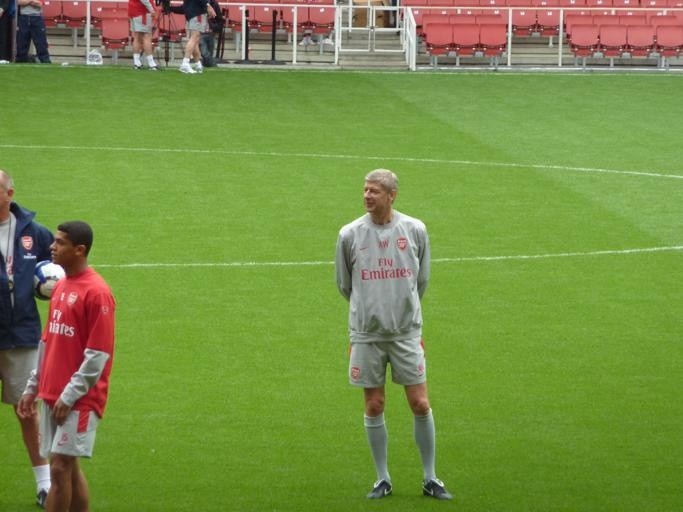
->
left=367, top=479, right=392, bottom=499
left=36, top=490, right=47, bottom=508
left=134, top=64, right=145, bottom=70
left=421, top=479, right=452, bottom=500
left=149, top=64, right=160, bottom=70
left=197, top=67, right=204, bottom=74
left=178, top=66, right=197, bottom=73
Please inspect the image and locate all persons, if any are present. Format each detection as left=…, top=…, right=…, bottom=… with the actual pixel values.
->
left=178, top=0, right=217, bottom=74
left=0, top=0, right=12, bottom=59
left=15, top=220, right=117, bottom=512
left=0, top=168, right=56, bottom=508
left=170, top=0, right=224, bottom=66
left=128, top=0, right=162, bottom=71
left=334, top=167, right=453, bottom=500
left=15, top=0, right=52, bottom=63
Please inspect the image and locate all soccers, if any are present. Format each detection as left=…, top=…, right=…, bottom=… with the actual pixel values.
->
left=31, top=260, right=66, bottom=299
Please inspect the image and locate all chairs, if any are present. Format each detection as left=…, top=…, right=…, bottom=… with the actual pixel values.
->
left=36, top=1, right=682, bottom=66
